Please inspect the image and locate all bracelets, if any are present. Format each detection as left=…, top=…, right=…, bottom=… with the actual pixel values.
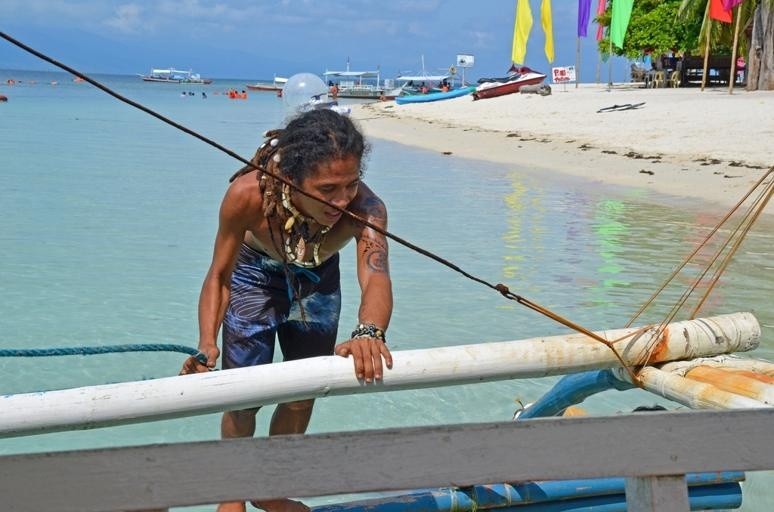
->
left=351, top=323, right=386, bottom=343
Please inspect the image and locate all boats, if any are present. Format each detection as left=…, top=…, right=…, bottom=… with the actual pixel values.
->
left=473, top=65, right=546, bottom=99
left=385, top=75, right=449, bottom=97
left=395, top=87, right=475, bottom=104
left=248, top=77, right=288, bottom=91
left=143, top=69, right=213, bottom=84
left=323, top=71, right=383, bottom=98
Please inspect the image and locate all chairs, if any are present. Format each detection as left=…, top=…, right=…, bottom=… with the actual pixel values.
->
left=643, top=68, right=681, bottom=87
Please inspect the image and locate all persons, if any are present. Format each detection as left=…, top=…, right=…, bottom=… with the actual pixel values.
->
left=226, top=87, right=247, bottom=100
left=180, top=90, right=207, bottom=100
left=417, top=78, right=450, bottom=93
left=181, top=110, right=393, bottom=512
left=331, top=82, right=339, bottom=100
